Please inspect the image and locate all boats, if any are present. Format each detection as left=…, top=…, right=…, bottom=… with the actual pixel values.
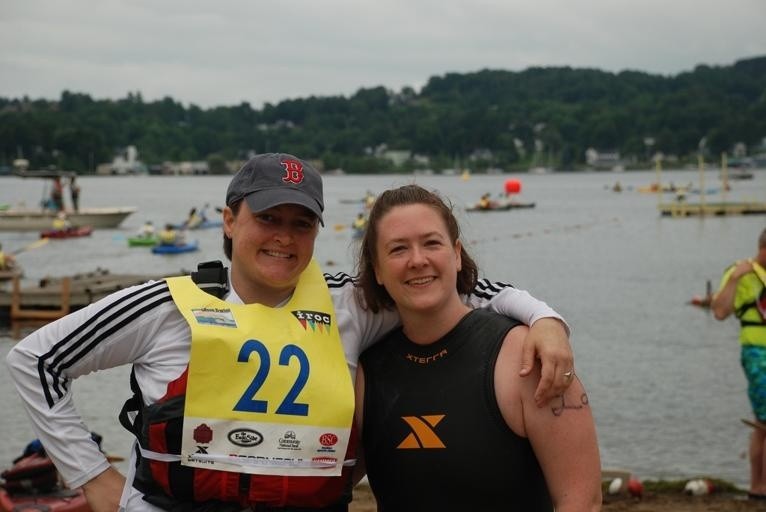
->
left=128, top=238, right=202, bottom=253
left=467, top=202, right=535, bottom=213
left=0, top=205, right=137, bottom=240
left=354, top=229, right=369, bottom=235
left=638, top=188, right=722, bottom=196
left=167, top=223, right=230, bottom=230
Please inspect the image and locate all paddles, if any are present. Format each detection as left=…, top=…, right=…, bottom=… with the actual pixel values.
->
left=9, top=237, right=49, bottom=256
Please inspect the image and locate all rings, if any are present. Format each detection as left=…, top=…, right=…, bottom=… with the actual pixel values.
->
left=564, top=372, right=572, bottom=379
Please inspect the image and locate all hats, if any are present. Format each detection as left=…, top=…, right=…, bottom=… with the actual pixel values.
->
left=226, top=153, right=324, bottom=227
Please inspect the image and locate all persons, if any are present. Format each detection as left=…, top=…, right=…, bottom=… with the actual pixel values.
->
left=51, top=177, right=65, bottom=206
left=138, top=199, right=213, bottom=248
left=351, top=184, right=605, bottom=512
left=352, top=188, right=379, bottom=233
left=3, top=152, right=576, bottom=512
left=68, top=170, right=83, bottom=207
left=709, top=225, right=766, bottom=501
